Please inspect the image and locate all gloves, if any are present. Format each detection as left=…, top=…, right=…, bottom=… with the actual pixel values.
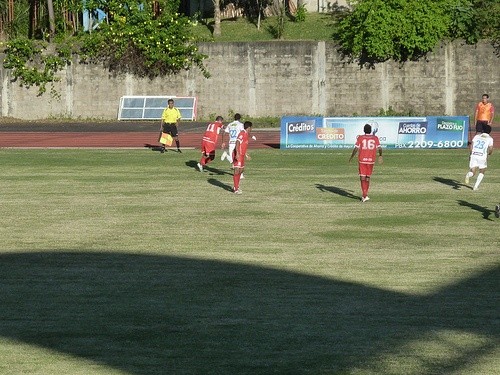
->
left=252, top=135, right=256, bottom=140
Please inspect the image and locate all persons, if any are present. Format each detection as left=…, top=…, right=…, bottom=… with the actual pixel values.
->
left=221, top=113, right=256, bottom=179
left=476, top=94, right=495, bottom=134
left=159, top=99, right=182, bottom=153
left=349, top=124, right=383, bottom=202
left=232, top=121, right=253, bottom=194
left=195, top=116, right=225, bottom=172
left=465, top=125, right=494, bottom=191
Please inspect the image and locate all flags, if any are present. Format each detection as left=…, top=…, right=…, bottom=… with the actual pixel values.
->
left=160, top=132, right=174, bottom=147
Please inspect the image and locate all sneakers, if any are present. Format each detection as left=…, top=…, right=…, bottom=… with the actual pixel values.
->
left=233, top=189, right=242, bottom=194
left=240, top=173, right=244, bottom=179
left=473, top=187, right=478, bottom=191
left=197, top=163, right=203, bottom=173
left=220, top=151, right=226, bottom=161
left=465, top=174, right=469, bottom=184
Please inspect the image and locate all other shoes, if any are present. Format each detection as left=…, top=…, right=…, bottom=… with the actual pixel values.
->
left=362, top=195, right=370, bottom=202
left=161, top=148, right=165, bottom=153
left=178, top=149, right=182, bottom=153
